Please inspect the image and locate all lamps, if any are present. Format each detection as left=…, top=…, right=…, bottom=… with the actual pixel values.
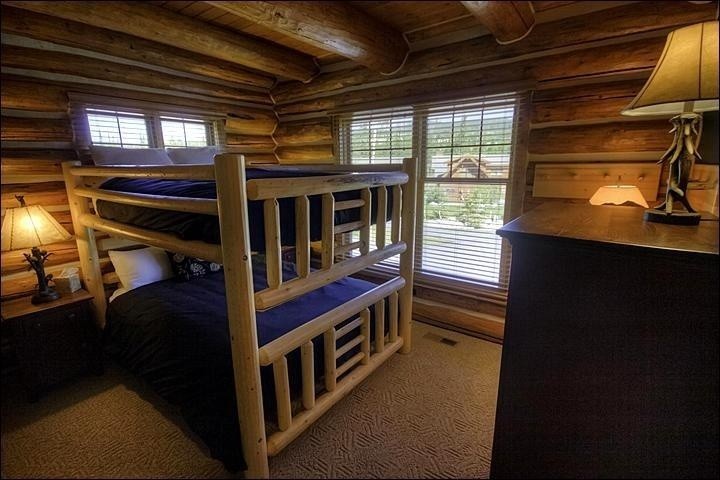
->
left=1, top=202, right=72, bottom=300
left=618, top=22, right=720, bottom=227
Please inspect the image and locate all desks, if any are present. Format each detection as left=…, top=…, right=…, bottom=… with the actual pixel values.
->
left=491, top=202, right=719, bottom=477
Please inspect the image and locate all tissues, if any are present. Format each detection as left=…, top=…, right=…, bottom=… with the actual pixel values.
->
left=52, top=266, right=81, bottom=294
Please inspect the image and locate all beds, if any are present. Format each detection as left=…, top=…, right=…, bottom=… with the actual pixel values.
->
left=60, top=157, right=417, bottom=479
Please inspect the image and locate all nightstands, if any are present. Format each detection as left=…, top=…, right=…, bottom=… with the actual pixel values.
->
left=2, top=289, right=105, bottom=399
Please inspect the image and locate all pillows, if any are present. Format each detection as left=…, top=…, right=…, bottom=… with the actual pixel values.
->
left=88, top=136, right=220, bottom=180
left=105, top=240, right=220, bottom=292
